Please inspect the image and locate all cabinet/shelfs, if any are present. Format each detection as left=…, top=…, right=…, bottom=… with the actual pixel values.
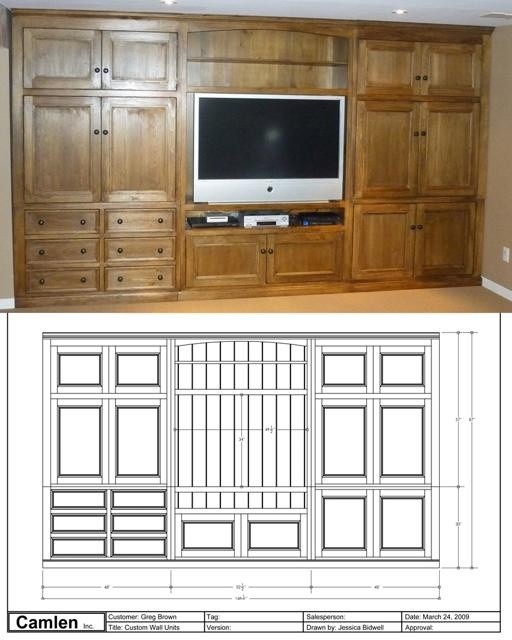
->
left=8, top=7, right=497, bottom=309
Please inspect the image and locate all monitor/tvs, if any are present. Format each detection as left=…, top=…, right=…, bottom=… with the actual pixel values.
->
left=193, top=91, right=346, bottom=206
